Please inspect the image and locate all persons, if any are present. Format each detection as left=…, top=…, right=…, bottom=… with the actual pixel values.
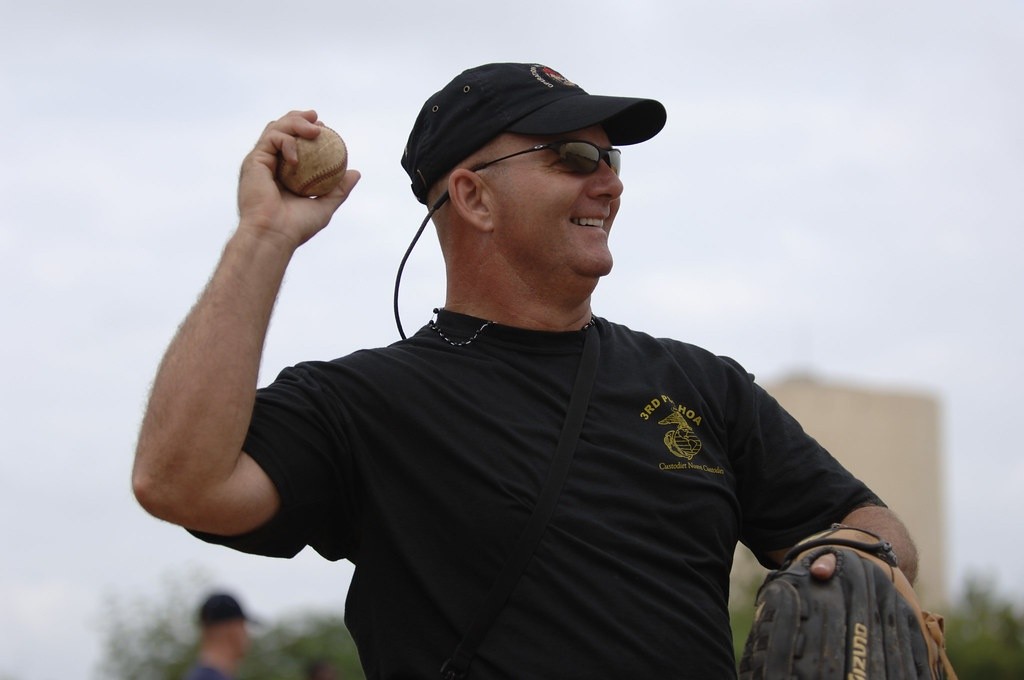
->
left=180, top=595, right=246, bottom=680
left=132, top=63, right=919, bottom=679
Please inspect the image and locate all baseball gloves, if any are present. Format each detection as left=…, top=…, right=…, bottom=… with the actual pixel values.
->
left=737, top=523, right=959, bottom=680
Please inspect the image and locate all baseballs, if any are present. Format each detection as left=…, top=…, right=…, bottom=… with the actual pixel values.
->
left=276, top=124, right=348, bottom=198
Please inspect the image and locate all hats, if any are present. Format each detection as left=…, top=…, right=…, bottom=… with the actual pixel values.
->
left=401, top=62, right=667, bottom=204
left=199, top=594, right=255, bottom=624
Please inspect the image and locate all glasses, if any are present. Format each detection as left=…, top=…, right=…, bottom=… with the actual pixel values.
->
left=473, top=139, right=622, bottom=179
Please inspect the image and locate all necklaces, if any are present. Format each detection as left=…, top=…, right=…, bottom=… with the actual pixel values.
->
left=430, top=312, right=596, bottom=347
